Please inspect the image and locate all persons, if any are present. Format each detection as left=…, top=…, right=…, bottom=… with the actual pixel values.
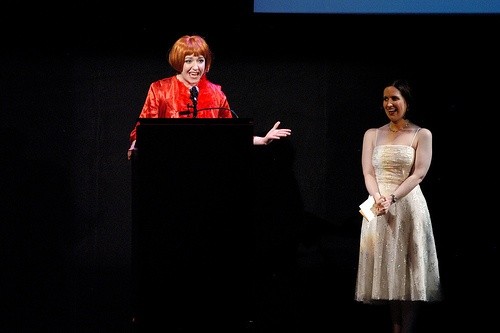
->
left=355, top=81, right=440, bottom=333
left=127, top=36, right=291, bottom=160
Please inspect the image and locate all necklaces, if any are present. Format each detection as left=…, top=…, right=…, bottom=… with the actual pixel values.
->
left=390, top=119, right=408, bottom=137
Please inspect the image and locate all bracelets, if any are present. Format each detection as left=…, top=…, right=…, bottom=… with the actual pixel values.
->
left=373, top=192, right=381, bottom=201
left=390, top=193, right=397, bottom=204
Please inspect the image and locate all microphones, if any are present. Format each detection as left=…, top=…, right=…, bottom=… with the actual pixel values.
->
left=189, top=86, right=198, bottom=95
left=179, top=107, right=238, bottom=118
